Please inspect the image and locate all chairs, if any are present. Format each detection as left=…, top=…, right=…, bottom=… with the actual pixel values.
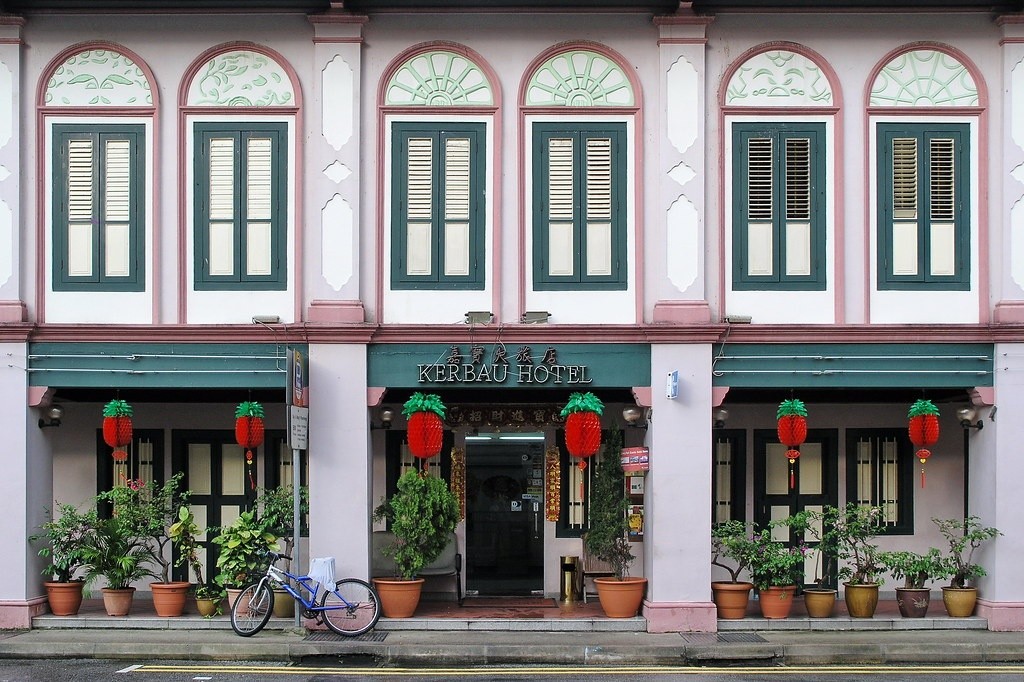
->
left=582, top=536, right=619, bottom=604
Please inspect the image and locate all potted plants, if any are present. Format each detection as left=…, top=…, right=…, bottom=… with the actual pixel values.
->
left=370, top=467, right=463, bottom=618
left=931, top=515, right=1005, bottom=618
left=768, top=507, right=850, bottom=618
left=712, top=519, right=767, bottom=619
left=92, top=471, right=191, bottom=618
left=581, top=454, right=648, bottom=618
left=77, top=526, right=163, bottom=616
left=26, top=499, right=98, bottom=616
left=168, top=507, right=221, bottom=618
left=747, top=539, right=815, bottom=619
left=876, top=546, right=950, bottom=618
left=254, top=481, right=309, bottom=619
left=209, top=510, right=281, bottom=617
left=822, top=499, right=897, bottom=619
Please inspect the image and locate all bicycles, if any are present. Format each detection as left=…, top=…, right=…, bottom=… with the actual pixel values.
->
left=230, top=540, right=381, bottom=637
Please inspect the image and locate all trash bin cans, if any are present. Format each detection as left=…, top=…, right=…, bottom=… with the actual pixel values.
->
left=560, top=556, right=579, bottom=602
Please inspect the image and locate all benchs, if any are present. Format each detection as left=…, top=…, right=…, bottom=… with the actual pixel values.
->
left=371, top=531, right=462, bottom=607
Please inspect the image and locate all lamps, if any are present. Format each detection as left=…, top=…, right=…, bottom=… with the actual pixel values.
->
left=38, top=404, right=64, bottom=430
left=622, top=404, right=648, bottom=429
left=712, top=405, right=730, bottom=429
left=955, top=407, right=983, bottom=429
left=370, top=407, right=394, bottom=431
